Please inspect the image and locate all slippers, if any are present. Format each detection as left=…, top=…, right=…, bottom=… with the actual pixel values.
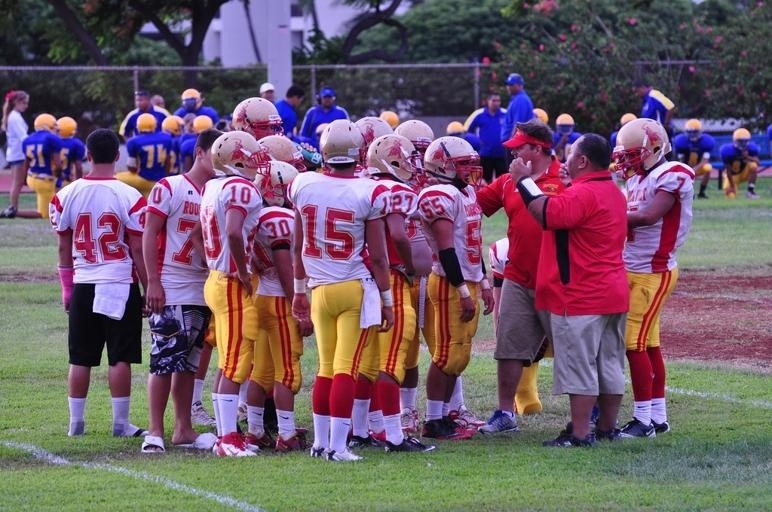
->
left=175, top=431, right=217, bottom=449
left=140, top=435, right=167, bottom=454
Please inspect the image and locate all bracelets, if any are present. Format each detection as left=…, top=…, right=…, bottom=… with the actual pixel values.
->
left=516, top=175, right=544, bottom=208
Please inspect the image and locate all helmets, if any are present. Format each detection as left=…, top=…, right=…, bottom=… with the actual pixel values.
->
left=319, top=112, right=483, bottom=186
left=488, top=238, right=510, bottom=276
left=686, top=119, right=700, bottom=131
left=612, top=118, right=673, bottom=180
left=321, top=86, right=336, bottom=97
left=34, top=113, right=77, bottom=138
left=212, top=97, right=307, bottom=206
left=182, top=90, right=203, bottom=110
left=734, top=127, right=752, bottom=142
left=504, top=74, right=525, bottom=85
left=137, top=113, right=213, bottom=136
left=557, top=114, right=574, bottom=136
left=532, top=108, right=549, bottom=124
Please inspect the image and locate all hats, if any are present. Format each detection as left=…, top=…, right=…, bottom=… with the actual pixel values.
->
left=501, top=127, right=553, bottom=149
left=259, top=83, right=274, bottom=93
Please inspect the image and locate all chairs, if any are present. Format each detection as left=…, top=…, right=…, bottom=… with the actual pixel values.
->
left=707, top=134, right=732, bottom=196
left=752, top=132, right=771, bottom=174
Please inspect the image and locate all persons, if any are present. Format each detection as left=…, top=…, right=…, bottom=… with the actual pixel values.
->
left=51, top=127, right=149, bottom=442
left=510, top=133, right=628, bottom=449
left=476, top=119, right=574, bottom=438
left=139, top=95, right=496, bottom=461
left=1, top=73, right=771, bottom=221
left=487, top=235, right=562, bottom=412
left=613, top=119, right=692, bottom=441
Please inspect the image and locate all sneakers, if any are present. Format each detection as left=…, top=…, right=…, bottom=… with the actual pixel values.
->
left=212, top=405, right=486, bottom=461
left=542, top=404, right=670, bottom=448
left=478, top=410, right=518, bottom=434
left=2, top=205, right=17, bottom=218
left=191, top=400, right=217, bottom=426
